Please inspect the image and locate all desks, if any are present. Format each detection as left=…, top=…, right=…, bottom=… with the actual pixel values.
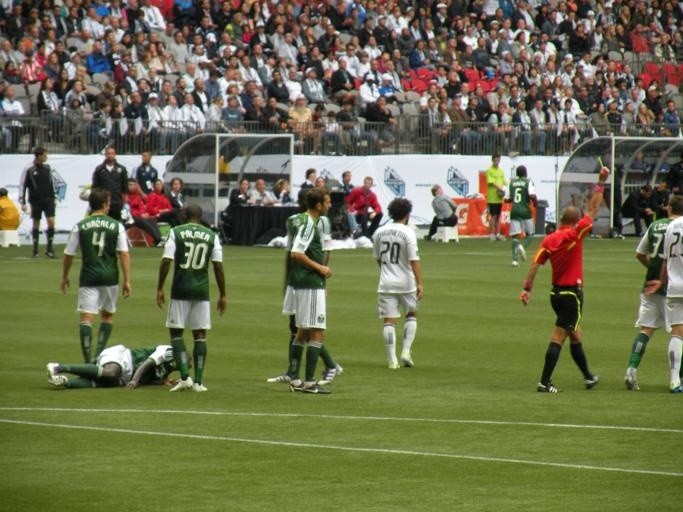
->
left=236, top=206, right=364, bottom=244
left=454, top=199, right=536, bottom=239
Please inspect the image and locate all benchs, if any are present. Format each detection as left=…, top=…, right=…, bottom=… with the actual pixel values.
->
left=593, top=214, right=634, bottom=235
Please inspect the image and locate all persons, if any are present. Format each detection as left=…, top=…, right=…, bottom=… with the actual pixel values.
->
left=45, top=343, right=194, bottom=389
left=621, top=194, right=683, bottom=392
left=519, top=164, right=610, bottom=393
left=230, top=179, right=293, bottom=204
left=506, top=165, right=537, bottom=266
left=1, top=0, right=683, bottom=156
left=1, top=147, right=67, bottom=257
left=289, top=188, right=333, bottom=394
left=92, top=146, right=186, bottom=247
left=59, top=188, right=134, bottom=363
left=658, top=215, right=683, bottom=393
left=595, top=151, right=683, bottom=237
left=297, top=168, right=354, bottom=190
left=346, top=177, right=382, bottom=238
left=156, top=204, right=227, bottom=392
left=486, top=153, right=506, bottom=241
left=426, top=185, right=458, bottom=240
left=266, top=188, right=345, bottom=383
left=372, top=197, right=424, bottom=371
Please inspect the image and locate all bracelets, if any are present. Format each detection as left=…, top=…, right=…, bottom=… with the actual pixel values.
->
left=522, top=278, right=532, bottom=292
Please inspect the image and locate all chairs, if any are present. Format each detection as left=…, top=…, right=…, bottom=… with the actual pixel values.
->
left=0, top=34, right=682, bottom=153
left=115, top=210, right=231, bottom=248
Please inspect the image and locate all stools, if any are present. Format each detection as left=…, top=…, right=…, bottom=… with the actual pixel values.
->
left=437, top=226, right=459, bottom=243
left=0, top=230, right=18, bottom=247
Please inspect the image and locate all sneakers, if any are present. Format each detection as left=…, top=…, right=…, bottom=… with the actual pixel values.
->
left=192, top=383, right=208, bottom=392
left=669, top=382, right=682, bottom=393
left=47, top=375, right=67, bottom=387
left=585, top=375, right=599, bottom=389
left=46, top=362, right=58, bottom=378
left=267, top=364, right=343, bottom=394
left=388, top=362, right=400, bottom=370
left=400, top=353, right=414, bottom=367
left=169, top=377, right=193, bottom=392
left=624, top=374, right=640, bottom=391
left=537, top=382, right=562, bottom=393
left=517, top=244, right=527, bottom=261
